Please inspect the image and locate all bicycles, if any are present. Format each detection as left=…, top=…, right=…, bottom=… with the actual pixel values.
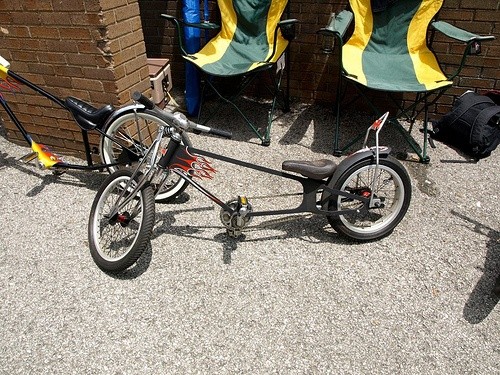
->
left=86, top=92, right=412, bottom=275
left=0, top=26, right=197, bottom=205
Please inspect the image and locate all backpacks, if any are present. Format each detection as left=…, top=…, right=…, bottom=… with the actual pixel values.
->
left=432, top=88, right=499, bottom=161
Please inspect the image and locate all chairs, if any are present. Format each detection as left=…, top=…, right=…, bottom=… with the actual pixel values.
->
left=160, top=0, right=299, bottom=147
left=316, top=0, right=496, bottom=165
